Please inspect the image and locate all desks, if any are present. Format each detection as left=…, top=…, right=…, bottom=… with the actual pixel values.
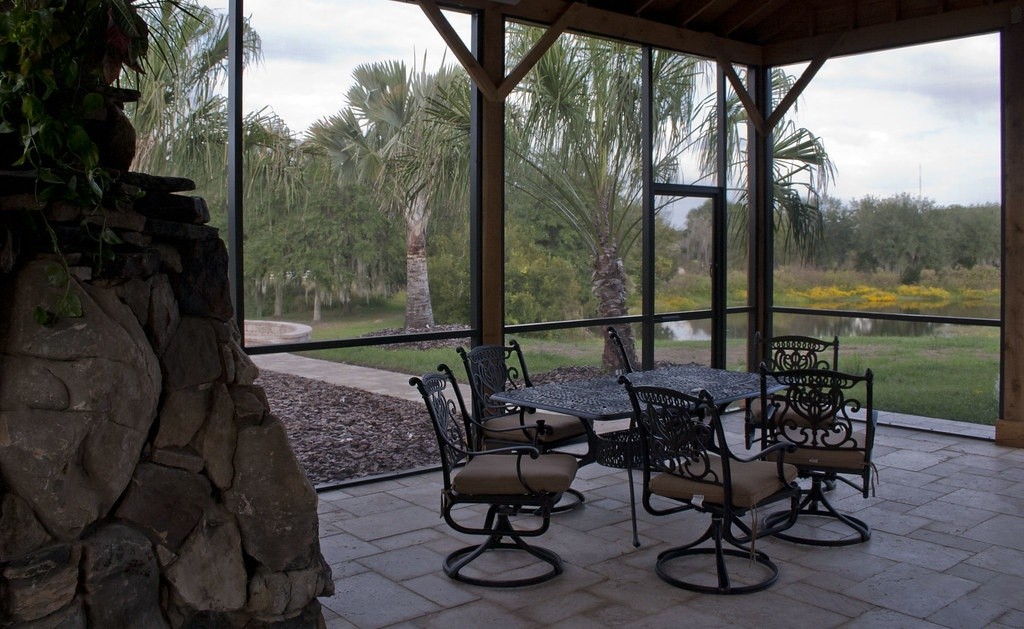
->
left=492, top=360, right=802, bottom=552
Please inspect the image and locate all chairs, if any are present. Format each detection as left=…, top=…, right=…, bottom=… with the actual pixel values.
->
left=407, top=328, right=878, bottom=597
